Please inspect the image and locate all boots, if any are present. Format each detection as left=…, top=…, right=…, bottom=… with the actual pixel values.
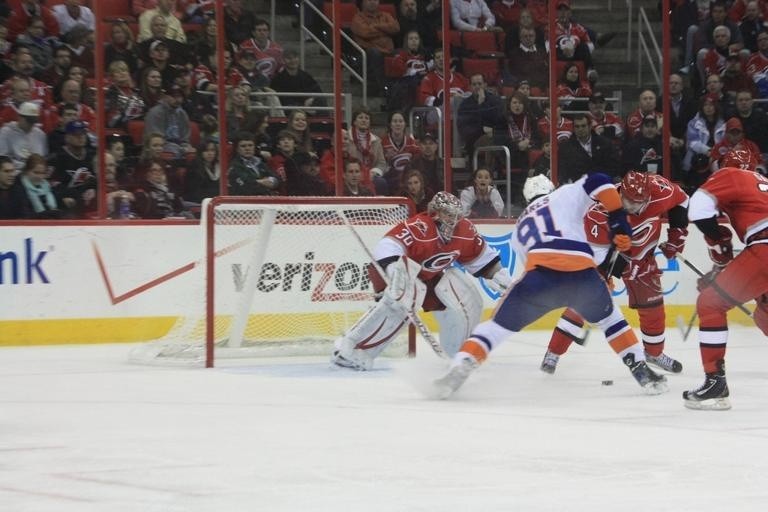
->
left=426, top=361, right=469, bottom=399
left=684, top=360, right=731, bottom=402
left=541, top=348, right=558, bottom=372
left=643, top=351, right=683, bottom=374
left=621, top=353, right=667, bottom=388
left=331, top=347, right=371, bottom=371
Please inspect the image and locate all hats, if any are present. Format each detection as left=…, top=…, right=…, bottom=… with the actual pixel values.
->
left=10, top=100, right=40, bottom=118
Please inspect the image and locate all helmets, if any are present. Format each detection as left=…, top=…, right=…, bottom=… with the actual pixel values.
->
left=428, top=190, right=463, bottom=242
left=622, top=169, right=651, bottom=204
left=718, top=147, right=757, bottom=172
left=522, top=174, right=554, bottom=201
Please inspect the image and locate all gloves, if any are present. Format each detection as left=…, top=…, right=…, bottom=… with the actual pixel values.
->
left=665, top=228, right=688, bottom=258
left=696, top=264, right=726, bottom=292
left=607, top=207, right=632, bottom=251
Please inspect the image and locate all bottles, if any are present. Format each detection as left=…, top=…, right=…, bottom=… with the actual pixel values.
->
left=120, top=193, right=131, bottom=220
left=105, top=194, right=116, bottom=220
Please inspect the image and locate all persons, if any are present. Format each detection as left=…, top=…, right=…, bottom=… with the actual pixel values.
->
left=683, top=167, right=768, bottom=402
left=0, top=2, right=768, bottom=217
left=425, top=171, right=667, bottom=401
left=540, top=169, right=689, bottom=376
left=330, top=192, right=513, bottom=371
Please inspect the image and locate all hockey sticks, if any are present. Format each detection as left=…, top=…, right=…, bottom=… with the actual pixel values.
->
left=677, top=266, right=721, bottom=341
left=565, top=250, right=621, bottom=344
